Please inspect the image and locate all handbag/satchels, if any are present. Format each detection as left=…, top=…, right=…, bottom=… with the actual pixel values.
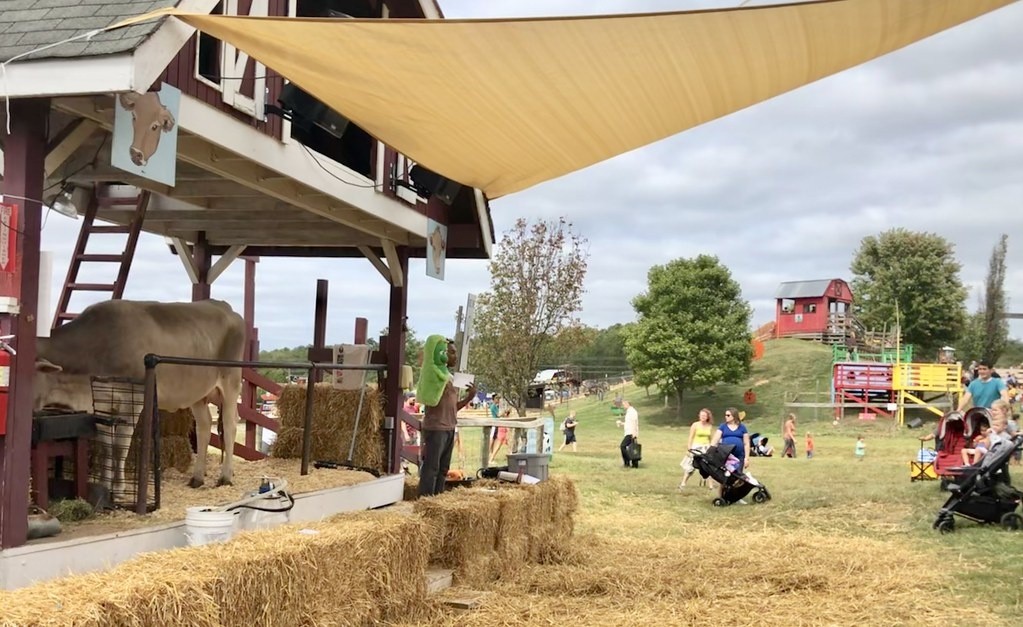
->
left=627, top=437, right=642, bottom=459
left=680, top=453, right=696, bottom=474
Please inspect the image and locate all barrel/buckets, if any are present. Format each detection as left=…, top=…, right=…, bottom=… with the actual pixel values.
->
left=184, top=507, right=234, bottom=547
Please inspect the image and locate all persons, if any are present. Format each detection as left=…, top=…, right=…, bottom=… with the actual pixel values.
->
left=919, top=360, right=1023, bottom=465
left=416, top=339, right=478, bottom=496
left=458, top=393, right=867, bottom=510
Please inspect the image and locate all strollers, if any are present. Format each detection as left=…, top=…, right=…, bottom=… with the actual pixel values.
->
left=932, top=405, right=1000, bottom=492
left=931, top=433, right=1023, bottom=537
left=685, top=444, right=772, bottom=507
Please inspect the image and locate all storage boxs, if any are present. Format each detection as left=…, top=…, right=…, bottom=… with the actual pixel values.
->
left=506, top=453, right=550, bottom=483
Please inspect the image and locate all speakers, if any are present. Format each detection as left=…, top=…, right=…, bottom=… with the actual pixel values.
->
left=410, top=163, right=460, bottom=205
left=275, top=10, right=361, bottom=139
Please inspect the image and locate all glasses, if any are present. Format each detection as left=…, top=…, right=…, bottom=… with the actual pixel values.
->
left=495, top=396, right=502, bottom=399
left=725, top=413, right=733, bottom=417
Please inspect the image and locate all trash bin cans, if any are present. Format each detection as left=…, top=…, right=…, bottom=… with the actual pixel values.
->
left=505, top=452, right=551, bottom=482
left=611, top=406, right=624, bottom=414
left=939, top=346, right=956, bottom=364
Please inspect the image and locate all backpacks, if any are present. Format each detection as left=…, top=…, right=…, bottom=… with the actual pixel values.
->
left=560, top=417, right=574, bottom=430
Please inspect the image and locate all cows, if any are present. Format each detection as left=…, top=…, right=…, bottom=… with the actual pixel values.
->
left=0, top=298, right=247, bottom=514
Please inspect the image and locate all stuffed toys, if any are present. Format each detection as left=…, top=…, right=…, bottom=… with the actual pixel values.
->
left=415, top=333, right=453, bottom=407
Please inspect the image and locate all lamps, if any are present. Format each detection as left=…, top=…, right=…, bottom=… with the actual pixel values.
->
left=44, top=180, right=77, bottom=219
